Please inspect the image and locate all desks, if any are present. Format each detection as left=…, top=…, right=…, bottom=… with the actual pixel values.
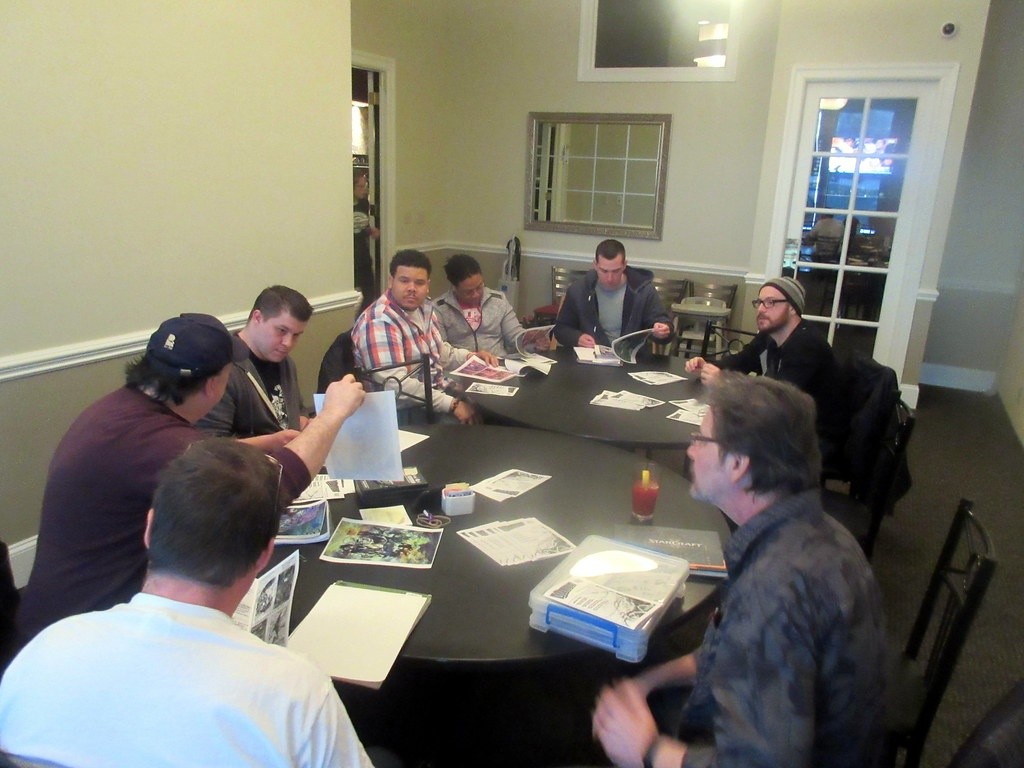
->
left=455, top=346, right=714, bottom=474
left=254, top=419, right=731, bottom=768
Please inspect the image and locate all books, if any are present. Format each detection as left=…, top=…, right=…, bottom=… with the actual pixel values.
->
left=614, top=522, right=729, bottom=577
left=231, top=548, right=300, bottom=648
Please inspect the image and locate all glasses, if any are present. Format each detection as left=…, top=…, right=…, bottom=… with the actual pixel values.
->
left=183, top=440, right=284, bottom=512
left=752, top=298, right=788, bottom=309
left=688, top=431, right=735, bottom=451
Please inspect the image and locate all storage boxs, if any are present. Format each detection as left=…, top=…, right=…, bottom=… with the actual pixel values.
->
left=528, top=535, right=690, bottom=662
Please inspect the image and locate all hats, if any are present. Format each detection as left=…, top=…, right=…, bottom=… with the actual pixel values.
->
left=146, top=313, right=250, bottom=377
left=758, top=276, right=806, bottom=318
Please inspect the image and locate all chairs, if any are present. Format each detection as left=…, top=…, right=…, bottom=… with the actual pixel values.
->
left=320, top=329, right=433, bottom=425
left=533, top=266, right=758, bottom=366
left=801, top=235, right=888, bottom=322
left=878, top=498, right=1024, bottom=768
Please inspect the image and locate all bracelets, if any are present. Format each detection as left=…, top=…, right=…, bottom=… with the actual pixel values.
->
left=452, top=399, right=461, bottom=415
left=641, top=735, right=670, bottom=768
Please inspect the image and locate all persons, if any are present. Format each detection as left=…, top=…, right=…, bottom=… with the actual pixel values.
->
left=802, top=208, right=884, bottom=335
left=0, top=432, right=378, bottom=767
left=553, top=239, right=674, bottom=358
left=353, top=170, right=381, bottom=322
left=432, top=252, right=551, bottom=370
left=16, top=313, right=366, bottom=655
left=593, top=370, right=905, bottom=768
left=351, top=248, right=500, bottom=426
left=189, top=284, right=313, bottom=454
left=684, top=276, right=854, bottom=536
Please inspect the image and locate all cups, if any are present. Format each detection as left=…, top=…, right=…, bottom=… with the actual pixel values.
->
left=440, top=488, right=476, bottom=516
left=630, top=462, right=661, bottom=523
